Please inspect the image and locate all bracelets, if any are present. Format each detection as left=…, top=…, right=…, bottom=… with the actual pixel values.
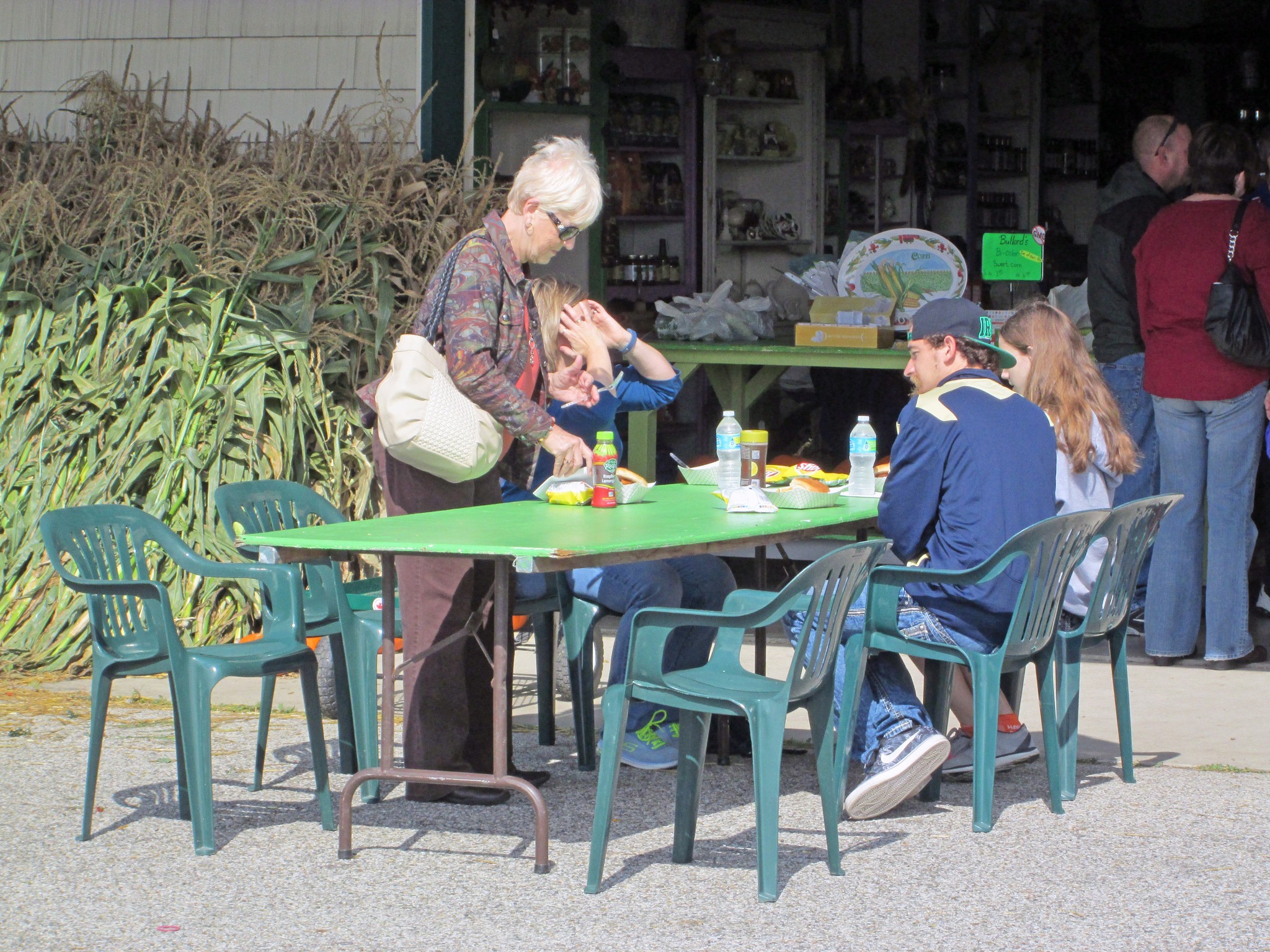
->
left=535, top=417, right=555, bottom=443
left=619, top=328, right=637, bottom=354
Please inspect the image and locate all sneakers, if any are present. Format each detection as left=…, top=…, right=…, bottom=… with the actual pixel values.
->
left=941, top=724, right=1041, bottom=779
left=845, top=720, right=951, bottom=821
left=595, top=709, right=680, bottom=770
left=653, top=722, right=681, bottom=748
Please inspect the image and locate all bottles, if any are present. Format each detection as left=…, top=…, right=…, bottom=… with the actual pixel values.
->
left=590, top=431, right=618, bottom=508
left=847, top=417, right=877, bottom=496
left=610, top=238, right=681, bottom=286
left=716, top=411, right=743, bottom=489
left=740, top=429, right=769, bottom=488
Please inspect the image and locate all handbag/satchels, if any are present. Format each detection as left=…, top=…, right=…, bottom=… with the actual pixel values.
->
left=1203, top=194, right=1270, bottom=368
left=373, top=235, right=508, bottom=485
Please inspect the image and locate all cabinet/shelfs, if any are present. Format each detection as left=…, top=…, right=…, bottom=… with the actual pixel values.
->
left=470, top=0, right=1114, bottom=454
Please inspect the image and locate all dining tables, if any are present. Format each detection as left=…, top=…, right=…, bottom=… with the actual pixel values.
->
left=227, top=466, right=897, bottom=876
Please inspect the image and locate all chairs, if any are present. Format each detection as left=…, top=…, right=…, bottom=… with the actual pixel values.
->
left=929, top=487, right=1189, bottom=801
left=826, top=504, right=1116, bottom=833
left=577, top=533, right=898, bottom=904
left=35, top=502, right=339, bottom=857
left=209, top=476, right=410, bottom=802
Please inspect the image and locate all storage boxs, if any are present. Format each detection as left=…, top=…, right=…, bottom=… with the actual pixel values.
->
left=793, top=295, right=896, bottom=348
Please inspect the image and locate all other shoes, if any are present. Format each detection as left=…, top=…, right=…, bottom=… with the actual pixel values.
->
left=1210, top=645, right=1268, bottom=671
left=1255, top=583, right=1270, bottom=612
left=1126, top=606, right=1145, bottom=637
left=1155, top=646, right=1198, bottom=666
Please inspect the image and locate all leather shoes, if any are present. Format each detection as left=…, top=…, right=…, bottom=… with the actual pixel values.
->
left=441, top=785, right=510, bottom=805
left=508, top=769, right=550, bottom=785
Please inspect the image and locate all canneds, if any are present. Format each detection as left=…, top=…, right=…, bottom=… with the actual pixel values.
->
left=620, top=254, right=681, bottom=286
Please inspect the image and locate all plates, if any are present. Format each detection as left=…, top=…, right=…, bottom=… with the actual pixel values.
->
left=836, top=228, right=968, bottom=330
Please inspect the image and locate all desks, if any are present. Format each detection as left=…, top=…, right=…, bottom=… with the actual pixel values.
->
left=619, top=332, right=910, bottom=490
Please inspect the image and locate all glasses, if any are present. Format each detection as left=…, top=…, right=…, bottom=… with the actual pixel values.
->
left=546, top=212, right=582, bottom=241
left=1156, top=116, right=1186, bottom=155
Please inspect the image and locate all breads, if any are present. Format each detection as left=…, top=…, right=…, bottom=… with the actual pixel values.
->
left=615, top=467, right=648, bottom=487
left=789, top=477, right=831, bottom=493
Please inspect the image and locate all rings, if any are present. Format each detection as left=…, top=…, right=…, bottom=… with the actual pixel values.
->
left=564, top=459, right=572, bottom=463
left=561, top=329, right=566, bottom=335
left=576, top=320, right=582, bottom=323
left=594, top=310, right=597, bottom=313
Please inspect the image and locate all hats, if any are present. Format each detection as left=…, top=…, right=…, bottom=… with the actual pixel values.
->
left=907, top=297, right=1016, bottom=368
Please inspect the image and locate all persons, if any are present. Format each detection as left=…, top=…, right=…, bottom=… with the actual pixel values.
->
left=779, top=297, right=1058, bottom=819
left=498, top=275, right=738, bottom=769
left=1132, top=120, right=1270, bottom=673
left=1087, top=114, right=1194, bottom=635
left=908, top=289, right=1142, bottom=774
left=353, top=134, right=602, bottom=806
